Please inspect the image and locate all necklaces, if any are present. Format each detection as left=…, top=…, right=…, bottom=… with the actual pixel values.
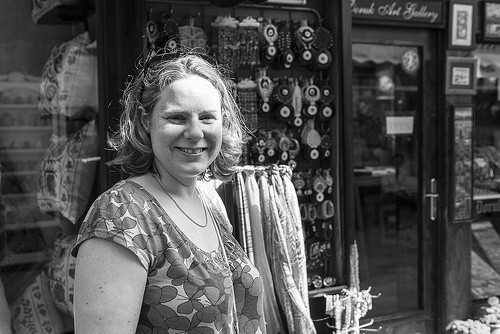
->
left=152, top=173, right=209, bottom=228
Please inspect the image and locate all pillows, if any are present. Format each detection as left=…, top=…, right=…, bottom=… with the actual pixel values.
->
left=9, top=271, right=69, bottom=334
left=47, top=230, right=77, bottom=319
left=39, top=31, right=92, bottom=118
left=35, top=120, right=100, bottom=224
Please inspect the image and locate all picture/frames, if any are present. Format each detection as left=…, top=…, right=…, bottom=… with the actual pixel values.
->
left=450, top=104, right=476, bottom=224
left=444, top=56, right=478, bottom=95
left=482, top=0, right=500, bottom=42
left=448, top=0, right=477, bottom=50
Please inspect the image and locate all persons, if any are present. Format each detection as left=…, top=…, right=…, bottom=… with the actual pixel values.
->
left=68, top=53, right=268, bottom=334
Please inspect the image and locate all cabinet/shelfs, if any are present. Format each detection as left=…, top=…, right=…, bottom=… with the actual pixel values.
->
left=0, top=73, right=64, bottom=267
